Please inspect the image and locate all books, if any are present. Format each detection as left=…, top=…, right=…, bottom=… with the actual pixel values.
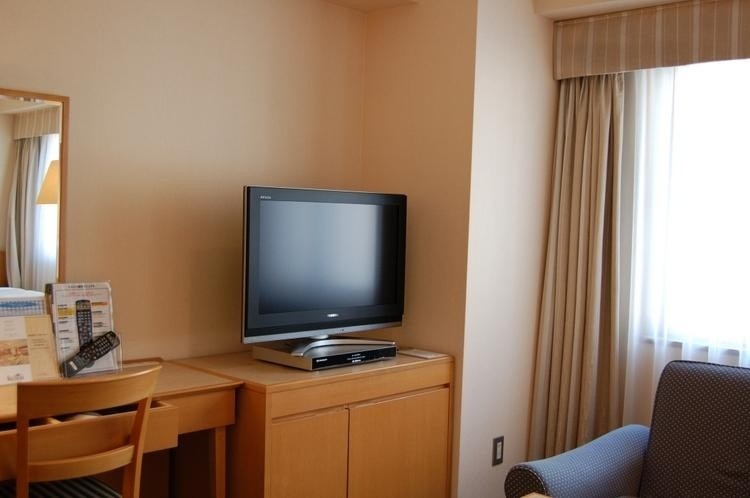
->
left=397, top=347, right=449, bottom=359
left=0, top=312, right=62, bottom=387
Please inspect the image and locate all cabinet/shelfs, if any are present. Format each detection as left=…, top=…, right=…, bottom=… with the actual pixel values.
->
left=263, top=360, right=453, bottom=498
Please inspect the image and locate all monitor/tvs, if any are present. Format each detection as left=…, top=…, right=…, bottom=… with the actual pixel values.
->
left=240, top=186, right=407, bottom=357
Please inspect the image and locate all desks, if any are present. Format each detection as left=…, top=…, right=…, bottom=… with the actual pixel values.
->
left=0, top=358, right=244, bottom=497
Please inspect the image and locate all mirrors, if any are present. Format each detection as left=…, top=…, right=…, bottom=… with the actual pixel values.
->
left=1, top=89, right=70, bottom=301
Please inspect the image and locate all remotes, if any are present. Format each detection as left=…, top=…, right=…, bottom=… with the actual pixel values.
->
left=60, top=331, right=120, bottom=377
left=76, top=300, right=95, bottom=368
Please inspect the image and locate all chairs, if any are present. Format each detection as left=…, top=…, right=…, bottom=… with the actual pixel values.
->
left=0, top=366, right=164, bottom=498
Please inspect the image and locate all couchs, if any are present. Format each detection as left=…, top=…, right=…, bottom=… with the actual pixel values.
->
left=504, top=357, right=748, bottom=498
